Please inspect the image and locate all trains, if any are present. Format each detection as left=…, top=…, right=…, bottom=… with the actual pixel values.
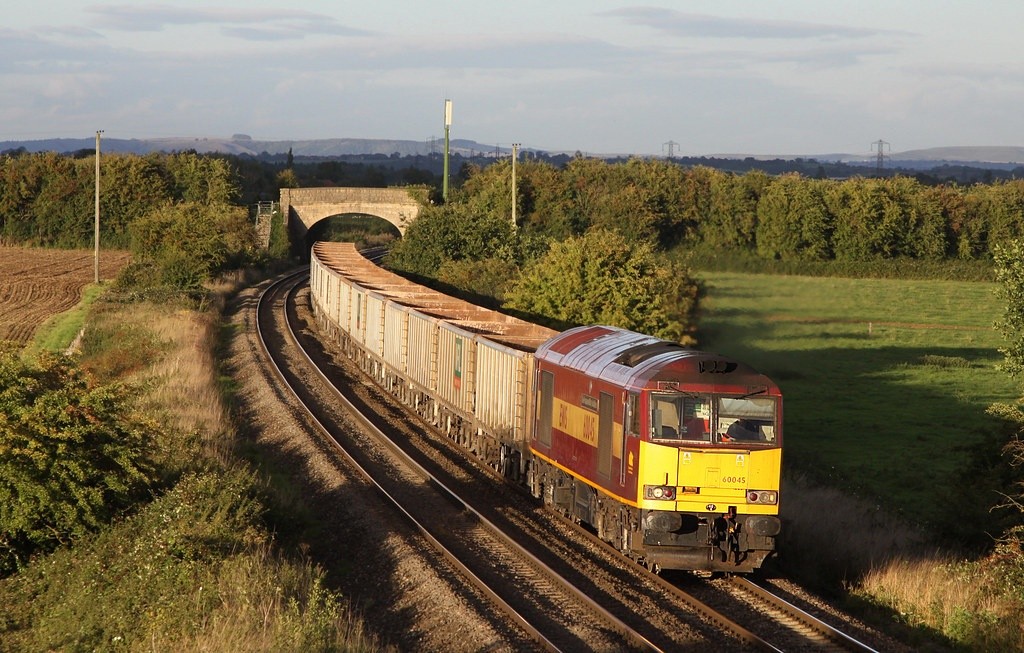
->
left=310, top=239, right=784, bottom=574
